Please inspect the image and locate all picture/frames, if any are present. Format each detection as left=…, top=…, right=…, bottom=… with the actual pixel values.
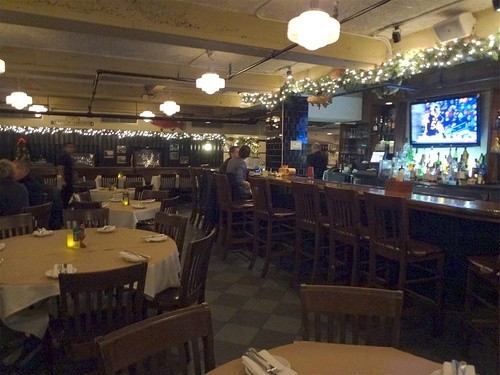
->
left=116, top=144, right=127, bottom=154
left=103, top=149, right=115, bottom=159
left=116, top=155, right=128, bottom=165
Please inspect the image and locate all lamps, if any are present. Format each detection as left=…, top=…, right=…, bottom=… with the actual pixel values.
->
left=285, top=65, right=294, bottom=80
left=287, top=0, right=341, bottom=51
left=493, top=0, right=500, bottom=13
left=28, top=104, right=48, bottom=118
left=391, top=25, right=402, bottom=43
left=0, top=59, right=6, bottom=74
left=5, top=76, right=33, bottom=111
left=195, top=49, right=226, bottom=95
left=159, top=90, right=181, bottom=117
left=140, top=110, right=156, bottom=123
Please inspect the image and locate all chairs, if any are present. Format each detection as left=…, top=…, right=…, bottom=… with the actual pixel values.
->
left=0, top=162, right=500, bottom=375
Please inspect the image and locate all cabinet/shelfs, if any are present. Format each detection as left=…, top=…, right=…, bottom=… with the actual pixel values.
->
left=370, top=100, right=408, bottom=153
left=339, top=122, right=371, bottom=170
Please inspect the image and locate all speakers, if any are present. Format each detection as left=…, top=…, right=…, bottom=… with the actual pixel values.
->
left=432, top=12, right=476, bottom=43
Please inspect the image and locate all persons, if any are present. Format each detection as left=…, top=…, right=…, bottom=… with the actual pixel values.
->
left=13, top=158, right=44, bottom=205
left=308, top=141, right=327, bottom=177
left=58, top=142, right=75, bottom=206
left=219, top=146, right=253, bottom=199
left=0, top=159, right=29, bottom=213
left=426, top=103, right=456, bottom=136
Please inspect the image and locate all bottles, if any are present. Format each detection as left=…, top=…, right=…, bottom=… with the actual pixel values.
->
left=493, top=109, right=500, bottom=136
left=337, top=114, right=396, bottom=173
left=395, top=137, right=500, bottom=186
left=66, top=219, right=86, bottom=248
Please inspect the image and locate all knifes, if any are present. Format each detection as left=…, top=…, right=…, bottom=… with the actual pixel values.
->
left=245, top=352, right=278, bottom=375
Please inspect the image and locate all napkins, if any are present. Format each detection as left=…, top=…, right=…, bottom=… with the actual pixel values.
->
left=118, top=251, right=151, bottom=262
left=143, top=233, right=168, bottom=243
left=32, top=227, right=48, bottom=237
left=53, top=261, right=73, bottom=279
left=442, top=361, right=477, bottom=375
left=96, top=224, right=116, bottom=234
left=240, top=348, right=298, bottom=375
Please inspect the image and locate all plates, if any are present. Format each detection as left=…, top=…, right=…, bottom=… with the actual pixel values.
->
left=97, top=225, right=116, bottom=232
left=145, top=234, right=167, bottom=241
left=100, top=198, right=154, bottom=208
left=33, top=230, right=54, bottom=236
left=245, top=355, right=291, bottom=375
left=45, top=266, right=77, bottom=278
left=125, top=253, right=150, bottom=261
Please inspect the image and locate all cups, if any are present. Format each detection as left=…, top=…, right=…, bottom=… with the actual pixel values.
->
left=306, top=166, right=314, bottom=180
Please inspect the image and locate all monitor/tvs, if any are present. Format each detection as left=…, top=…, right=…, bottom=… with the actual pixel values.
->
left=409, top=91, right=481, bottom=148
left=369, top=150, right=387, bottom=165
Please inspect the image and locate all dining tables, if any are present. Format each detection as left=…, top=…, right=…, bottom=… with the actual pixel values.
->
left=67, top=199, right=161, bottom=230
left=89, top=188, right=135, bottom=202
left=0, top=226, right=183, bottom=375
left=203, top=339, right=444, bottom=375
left=151, top=174, right=199, bottom=191
left=95, top=174, right=147, bottom=189
